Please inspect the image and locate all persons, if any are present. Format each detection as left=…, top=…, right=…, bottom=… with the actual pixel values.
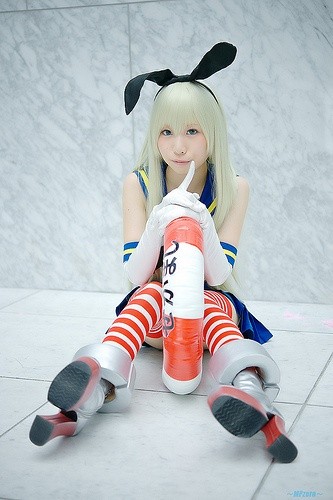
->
left=30, top=79, right=298, bottom=464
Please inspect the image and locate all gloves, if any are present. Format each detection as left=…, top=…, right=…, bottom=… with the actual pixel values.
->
left=125, top=160, right=205, bottom=287
left=157, top=194, right=232, bottom=287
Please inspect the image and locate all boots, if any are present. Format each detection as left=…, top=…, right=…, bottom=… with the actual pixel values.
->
left=207, top=368, right=298, bottom=464
left=30, top=355, right=109, bottom=446
